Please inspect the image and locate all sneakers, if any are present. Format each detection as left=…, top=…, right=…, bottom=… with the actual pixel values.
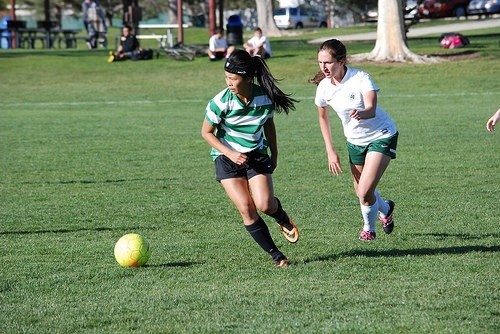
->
left=359, top=230, right=376, bottom=240
left=277, top=210, right=299, bottom=243
left=380, top=200, right=395, bottom=234
left=277, top=259, right=288, bottom=267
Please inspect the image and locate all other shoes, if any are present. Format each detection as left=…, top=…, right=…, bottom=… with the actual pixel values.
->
left=107, top=50, right=117, bottom=62
left=209, top=57, right=216, bottom=61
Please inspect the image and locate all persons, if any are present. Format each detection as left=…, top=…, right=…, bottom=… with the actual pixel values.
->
left=207, top=27, right=236, bottom=61
left=201, top=50, right=299, bottom=268
left=107, top=26, right=140, bottom=63
left=485, top=108, right=500, bottom=132
left=80, top=0, right=109, bottom=49
left=243, top=28, right=272, bottom=58
left=314, top=39, right=400, bottom=242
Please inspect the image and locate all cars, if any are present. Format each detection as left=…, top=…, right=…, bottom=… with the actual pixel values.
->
left=418, top=1, right=460, bottom=20
left=466, top=1, right=500, bottom=20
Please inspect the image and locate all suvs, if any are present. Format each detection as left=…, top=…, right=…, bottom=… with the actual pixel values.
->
left=271, top=6, right=328, bottom=32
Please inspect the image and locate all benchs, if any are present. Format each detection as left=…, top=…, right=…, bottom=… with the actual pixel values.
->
left=135, top=35, right=168, bottom=48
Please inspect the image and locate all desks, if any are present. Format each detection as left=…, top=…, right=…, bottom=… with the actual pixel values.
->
left=117, top=24, right=192, bottom=48
left=16, top=27, right=81, bottom=49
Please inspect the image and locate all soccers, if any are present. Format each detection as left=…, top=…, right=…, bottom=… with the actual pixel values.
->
left=113, top=234, right=151, bottom=268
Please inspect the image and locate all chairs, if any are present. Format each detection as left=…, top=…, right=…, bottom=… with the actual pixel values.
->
left=7, top=20, right=59, bottom=48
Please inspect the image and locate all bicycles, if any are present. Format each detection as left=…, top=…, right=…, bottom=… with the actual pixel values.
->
left=154, top=37, right=210, bottom=61
